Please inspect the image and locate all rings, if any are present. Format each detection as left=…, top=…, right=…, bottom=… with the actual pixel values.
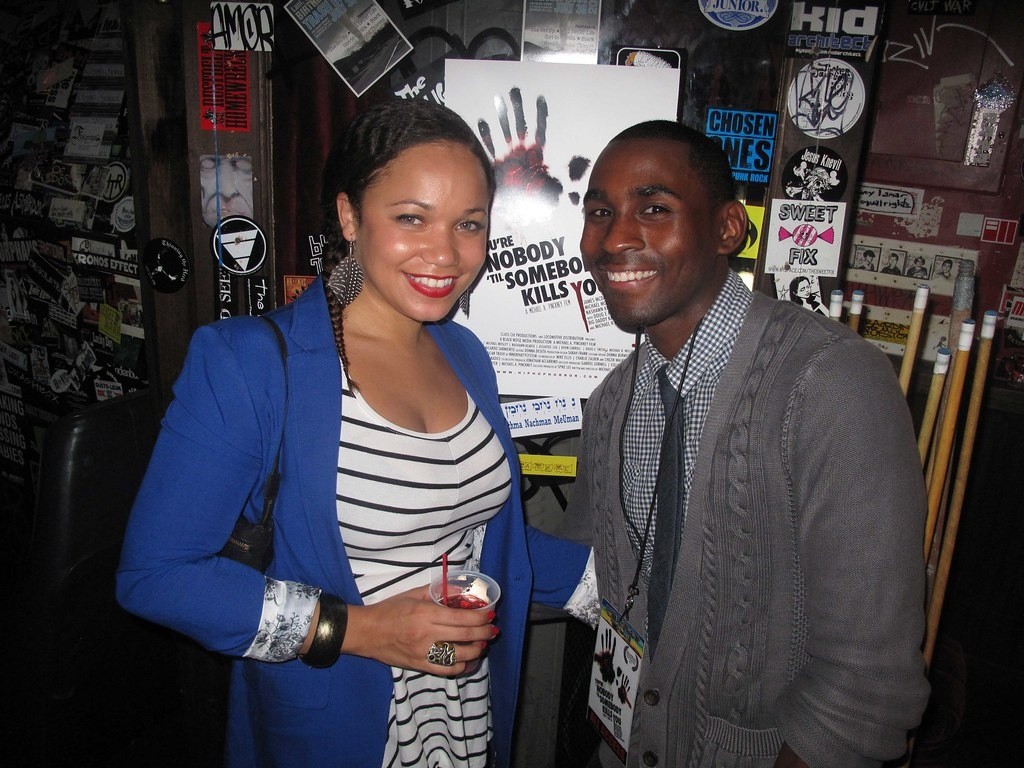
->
left=427, top=641, right=456, bottom=666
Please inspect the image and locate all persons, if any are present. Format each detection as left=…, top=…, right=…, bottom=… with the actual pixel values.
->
left=115, top=100, right=602, bottom=768
left=530, top=120, right=932, bottom=768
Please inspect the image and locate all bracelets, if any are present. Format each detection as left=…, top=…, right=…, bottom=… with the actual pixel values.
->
left=298, top=593, right=348, bottom=668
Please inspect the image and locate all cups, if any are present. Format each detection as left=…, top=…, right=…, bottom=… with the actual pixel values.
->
left=428, top=570, right=501, bottom=675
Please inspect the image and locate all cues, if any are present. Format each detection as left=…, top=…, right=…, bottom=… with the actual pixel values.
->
left=919, top=312, right=1000, bottom=667
left=829, top=288, right=844, bottom=322
left=899, top=283, right=931, bottom=396
left=850, top=292, right=864, bottom=332
